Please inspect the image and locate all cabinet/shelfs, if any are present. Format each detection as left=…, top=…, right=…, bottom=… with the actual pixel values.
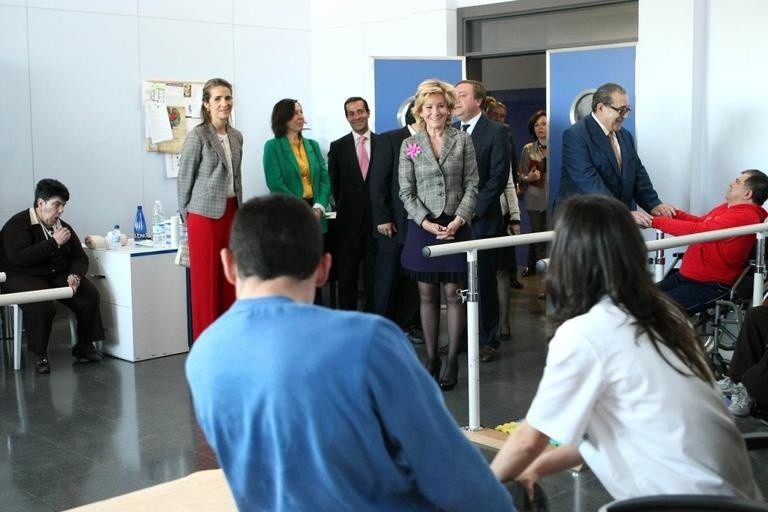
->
left=79, top=241, right=192, bottom=363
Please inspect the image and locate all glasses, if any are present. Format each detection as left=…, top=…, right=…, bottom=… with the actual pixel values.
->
left=609, top=105, right=632, bottom=118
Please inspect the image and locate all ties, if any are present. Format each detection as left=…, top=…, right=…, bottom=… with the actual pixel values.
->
left=462, top=125, right=470, bottom=131
left=359, top=136, right=369, bottom=181
left=609, top=133, right=622, bottom=169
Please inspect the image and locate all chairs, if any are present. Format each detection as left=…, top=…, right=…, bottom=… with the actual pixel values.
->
left=0, top=299, right=78, bottom=370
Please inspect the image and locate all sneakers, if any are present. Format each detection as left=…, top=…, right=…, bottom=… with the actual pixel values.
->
left=716, top=373, right=751, bottom=416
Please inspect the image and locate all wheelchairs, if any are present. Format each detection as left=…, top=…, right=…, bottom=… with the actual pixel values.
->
left=648, top=216, right=767, bottom=381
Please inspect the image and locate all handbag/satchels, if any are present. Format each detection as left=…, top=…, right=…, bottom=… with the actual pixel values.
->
left=528, top=160, right=544, bottom=187
left=175, top=240, right=191, bottom=268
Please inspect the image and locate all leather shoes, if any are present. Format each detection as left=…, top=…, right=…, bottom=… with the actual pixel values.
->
left=35, top=358, right=50, bottom=374
left=403, top=330, right=424, bottom=344
left=439, top=378, right=458, bottom=390
left=438, top=326, right=510, bottom=362
left=71, top=344, right=102, bottom=361
left=511, top=265, right=533, bottom=288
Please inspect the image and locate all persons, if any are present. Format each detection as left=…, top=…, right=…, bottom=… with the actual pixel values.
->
left=0, top=179, right=104, bottom=374
left=175, top=79, right=549, bottom=393
left=490, top=192, right=766, bottom=504
left=641, top=168, right=767, bottom=417
left=183, top=194, right=519, bottom=512
left=554, top=81, right=680, bottom=228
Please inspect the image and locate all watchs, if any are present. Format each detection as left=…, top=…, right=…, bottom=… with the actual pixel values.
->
left=67, top=274, right=81, bottom=281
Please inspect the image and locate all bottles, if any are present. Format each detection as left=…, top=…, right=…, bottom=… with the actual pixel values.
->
left=112, top=200, right=186, bottom=249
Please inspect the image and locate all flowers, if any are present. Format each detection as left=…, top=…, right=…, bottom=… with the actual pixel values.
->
left=406, top=142, right=423, bottom=160
